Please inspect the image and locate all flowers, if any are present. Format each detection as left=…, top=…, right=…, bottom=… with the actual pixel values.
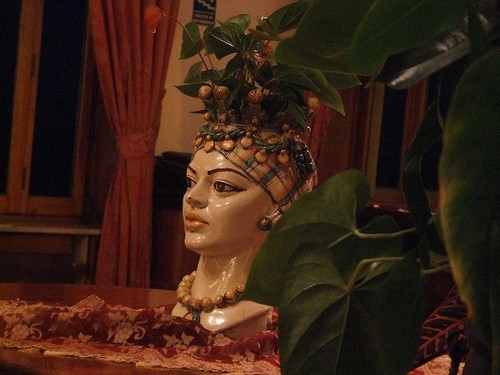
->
left=144, top=3, right=363, bottom=137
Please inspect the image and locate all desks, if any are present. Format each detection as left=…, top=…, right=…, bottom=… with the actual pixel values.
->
left=153, top=209, right=201, bottom=290
left=0, top=283, right=464, bottom=375
left=0, top=219, right=102, bottom=284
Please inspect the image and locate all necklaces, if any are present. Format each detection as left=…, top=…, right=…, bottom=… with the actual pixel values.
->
left=176, top=271, right=245, bottom=310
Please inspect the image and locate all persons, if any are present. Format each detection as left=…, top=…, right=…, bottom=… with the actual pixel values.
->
left=172, top=119, right=318, bottom=332
